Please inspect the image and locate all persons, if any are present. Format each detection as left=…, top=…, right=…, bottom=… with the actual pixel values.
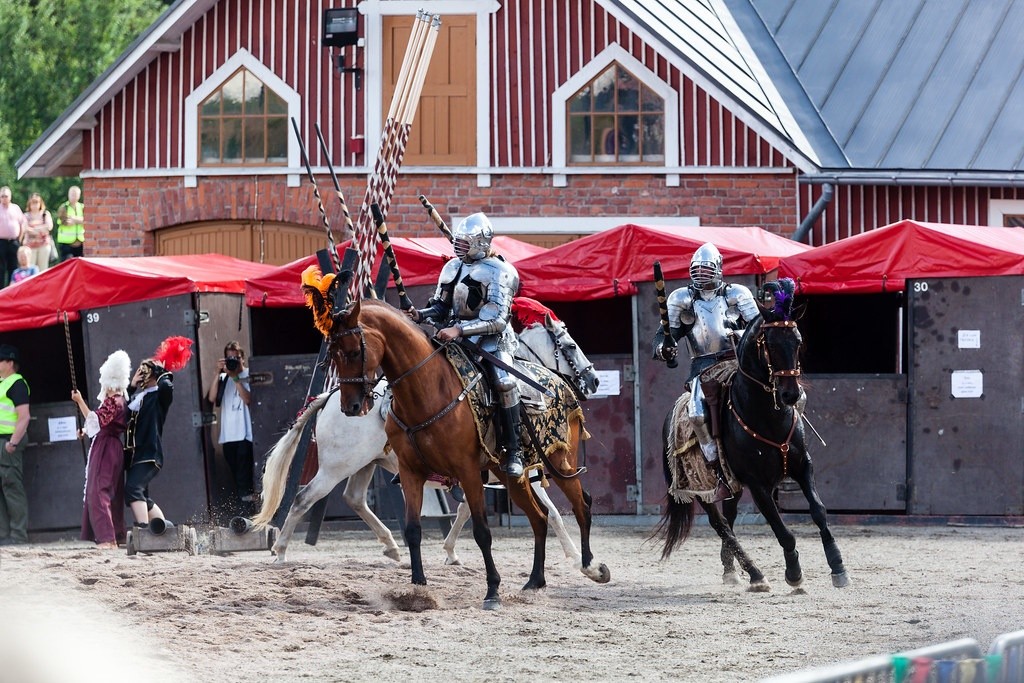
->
left=0, top=186, right=27, bottom=290
left=209, top=340, right=256, bottom=512
left=10, top=246, right=40, bottom=288
left=21, top=193, right=59, bottom=274
left=652, top=242, right=807, bottom=466
left=0, top=350, right=31, bottom=545
left=123, top=357, right=174, bottom=529
left=57, top=186, right=85, bottom=263
left=391, top=214, right=523, bottom=478
left=71, top=356, right=127, bottom=546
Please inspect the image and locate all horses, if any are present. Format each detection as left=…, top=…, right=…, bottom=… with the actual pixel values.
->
left=251, top=296, right=610, bottom=612
left=660, top=297, right=851, bottom=593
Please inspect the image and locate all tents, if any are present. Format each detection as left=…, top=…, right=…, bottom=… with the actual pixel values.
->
left=0, top=219, right=1024, bottom=331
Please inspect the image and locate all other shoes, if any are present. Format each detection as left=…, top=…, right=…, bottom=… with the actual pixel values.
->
left=0, top=537, right=23, bottom=547
left=699, top=435, right=718, bottom=461
left=505, top=451, right=524, bottom=476
left=96, top=540, right=117, bottom=549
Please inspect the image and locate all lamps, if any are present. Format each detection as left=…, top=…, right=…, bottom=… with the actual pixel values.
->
left=321, top=7, right=360, bottom=91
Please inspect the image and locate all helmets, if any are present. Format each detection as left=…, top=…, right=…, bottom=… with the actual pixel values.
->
left=687, top=241, right=723, bottom=285
left=454, top=212, right=494, bottom=260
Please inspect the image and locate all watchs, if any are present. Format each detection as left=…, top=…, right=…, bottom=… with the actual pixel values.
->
left=8, top=442, right=16, bottom=447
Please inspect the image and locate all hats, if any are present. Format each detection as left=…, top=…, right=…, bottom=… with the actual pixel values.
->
left=0, top=348, right=15, bottom=362
